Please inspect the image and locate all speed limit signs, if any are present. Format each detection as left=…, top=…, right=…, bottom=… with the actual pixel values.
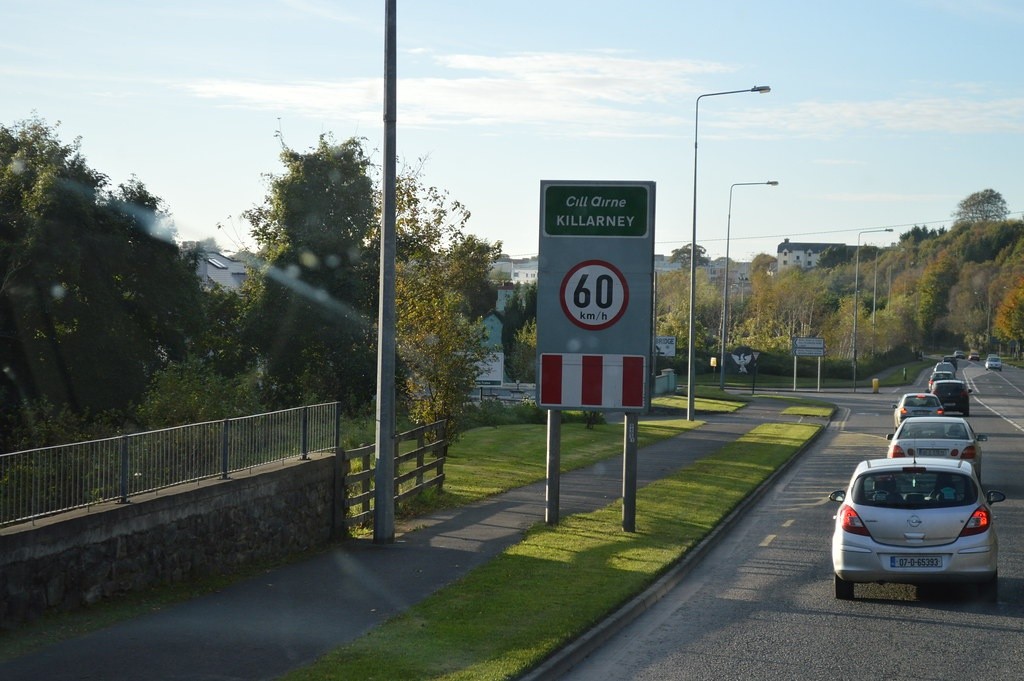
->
left=559, top=260, right=630, bottom=331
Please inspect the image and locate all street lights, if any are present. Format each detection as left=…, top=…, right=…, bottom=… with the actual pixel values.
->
left=686, top=85, right=773, bottom=419
left=719, top=181, right=779, bottom=390
left=851, top=229, right=894, bottom=393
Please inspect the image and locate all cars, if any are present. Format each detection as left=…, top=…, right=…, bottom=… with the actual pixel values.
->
left=984, top=356, right=1003, bottom=371
left=892, top=393, right=946, bottom=431
left=927, top=371, right=955, bottom=391
left=953, top=350, right=966, bottom=359
left=941, top=355, right=958, bottom=370
left=932, top=362, right=956, bottom=378
left=886, top=415, right=988, bottom=484
left=968, top=351, right=981, bottom=361
left=828, top=456, right=1007, bottom=601
left=925, top=379, right=973, bottom=417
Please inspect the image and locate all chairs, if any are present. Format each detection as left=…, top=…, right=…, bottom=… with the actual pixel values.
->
left=929, top=473, right=958, bottom=499
left=871, top=478, right=903, bottom=502
